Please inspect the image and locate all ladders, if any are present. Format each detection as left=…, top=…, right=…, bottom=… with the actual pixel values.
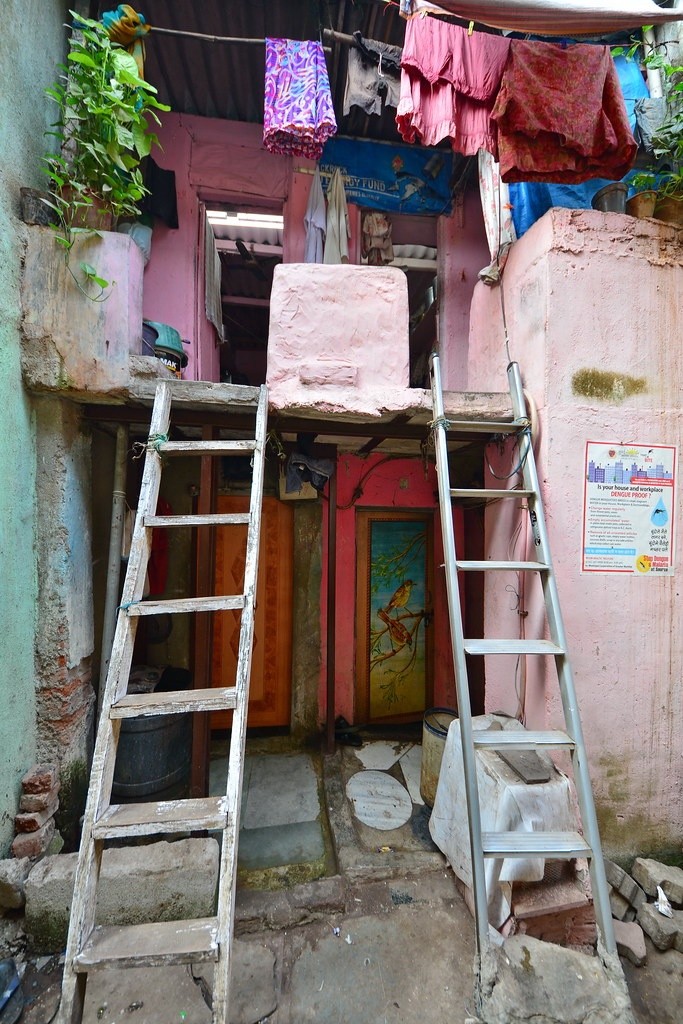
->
left=59, top=386, right=268, bottom=1024
left=430, top=354, right=621, bottom=981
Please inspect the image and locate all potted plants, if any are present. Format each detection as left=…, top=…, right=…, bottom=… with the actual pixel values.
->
left=17, top=184, right=54, bottom=228
left=18, top=6, right=171, bottom=305
left=589, top=24, right=683, bottom=223
